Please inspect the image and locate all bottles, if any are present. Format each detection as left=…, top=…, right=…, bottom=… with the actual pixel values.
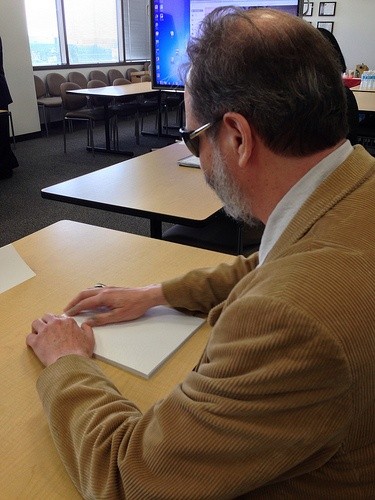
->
left=359, top=71, right=375, bottom=89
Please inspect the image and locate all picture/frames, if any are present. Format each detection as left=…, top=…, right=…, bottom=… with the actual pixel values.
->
left=319, top=2, right=336, bottom=16
left=303, top=2, right=313, bottom=16
left=317, top=21, right=334, bottom=34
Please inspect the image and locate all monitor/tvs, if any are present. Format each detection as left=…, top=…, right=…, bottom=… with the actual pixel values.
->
left=150, top=0, right=304, bottom=92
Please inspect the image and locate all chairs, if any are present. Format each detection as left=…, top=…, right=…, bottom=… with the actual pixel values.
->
left=33, top=62, right=169, bottom=158
left=350, top=116, right=375, bottom=145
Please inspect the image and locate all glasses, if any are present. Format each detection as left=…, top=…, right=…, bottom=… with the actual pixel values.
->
left=179, top=115, right=222, bottom=157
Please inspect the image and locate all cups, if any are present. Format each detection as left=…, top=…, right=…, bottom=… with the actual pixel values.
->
left=349, top=70, right=355, bottom=79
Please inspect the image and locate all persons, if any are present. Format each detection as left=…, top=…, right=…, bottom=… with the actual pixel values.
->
left=0, top=37, right=20, bottom=170
left=26, top=5, right=374, bottom=500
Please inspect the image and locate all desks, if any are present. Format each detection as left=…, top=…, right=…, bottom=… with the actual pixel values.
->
left=350, top=85, right=375, bottom=115
left=41, top=142, right=244, bottom=257
left=65, top=82, right=163, bottom=158
left=0, top=219, right=241, bottom=500
